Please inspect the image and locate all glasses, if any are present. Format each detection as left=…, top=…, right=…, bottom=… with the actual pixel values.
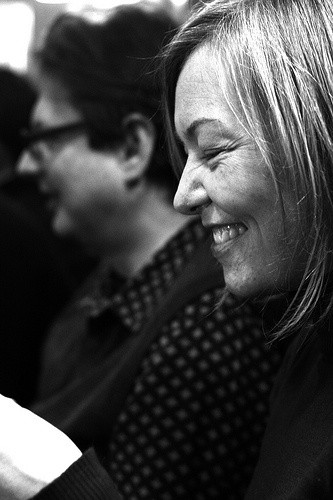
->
left=21, top=114, right=121, bottom=161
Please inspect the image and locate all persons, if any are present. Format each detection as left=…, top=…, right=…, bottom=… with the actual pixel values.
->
left=0, top=4, right=208, bottom=460
left=0, top=1, right=333, bottom=498
left=1, top=65, right=103, bottom=399
